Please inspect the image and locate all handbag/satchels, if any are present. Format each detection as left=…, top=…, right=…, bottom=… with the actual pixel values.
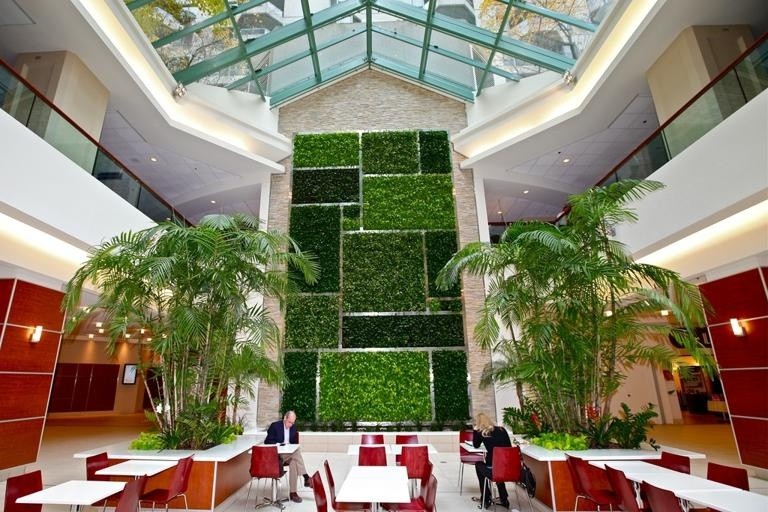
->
left=518, top=463, right=537, bottom=499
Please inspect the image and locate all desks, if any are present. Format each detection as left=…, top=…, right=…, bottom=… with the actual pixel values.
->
left=95, top=459, right=178, bottom=481
left=248, top=444, right=300, bottom=510
left=347, top=444, right=438, bottom=455
left=335, top=465, right=411, bottom=510
left=588, top=461, right=767, bottom=512
left=16, top=480, right=128, bottom=510
left=459, top=441, right=488, bottom=463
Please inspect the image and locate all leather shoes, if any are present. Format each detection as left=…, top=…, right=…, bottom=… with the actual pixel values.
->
left=304, top=477, right=313, bottom=487
left=290, top=492, right=302, bottom=502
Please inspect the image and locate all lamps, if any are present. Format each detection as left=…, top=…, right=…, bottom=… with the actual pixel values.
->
left=728, top=313, right=748, bottom=339
left=27, top=322, right=45, bottom=344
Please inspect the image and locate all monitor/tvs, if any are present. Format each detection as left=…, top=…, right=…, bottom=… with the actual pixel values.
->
left=122, top=363, right=138, bottom=385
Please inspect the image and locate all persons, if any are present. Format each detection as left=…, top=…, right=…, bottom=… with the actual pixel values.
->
left=473, top=412, right=512, bottom=509
left=264, top=411, right=313, bottom=503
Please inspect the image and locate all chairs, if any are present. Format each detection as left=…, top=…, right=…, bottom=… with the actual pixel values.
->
left=14, top=470, right=43, bottom=511
left=86, top=453, right=111, bottom=480
left=458, top=431, right=485, bottom=496
left=411, top=457, right=433, bottom=503
left=323, top=459, right=372, bottom=511
left=604, top=465, right=640, bottom=511
left=311, top=470, right=327, bottom=511
left=401, top=446, right=433, bottom=488
left=362, top=435, right=384, bottom=443
left=396, top=435, right=419, bottom=462
left=688, top=462, right=750, bottom=511
left=139, top=457, right=193, bottom=509
left=116, top=474, right=146, bottom=511
left=482, top=447, right=533, bottom=510
left=640, top=451, right=690, bottom=511
left=565, top=454, right=588, bottom=511
left=359, top=447, right=387, bottom=467
left=575, top=460, right=623, bottom=511
left=243, top=446, right=287, bottom=512
left=398, top=474, right=437, bottom=511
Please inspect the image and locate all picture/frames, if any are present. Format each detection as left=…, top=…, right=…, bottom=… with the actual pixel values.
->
left=121, top=362, right=138, bottom=387
